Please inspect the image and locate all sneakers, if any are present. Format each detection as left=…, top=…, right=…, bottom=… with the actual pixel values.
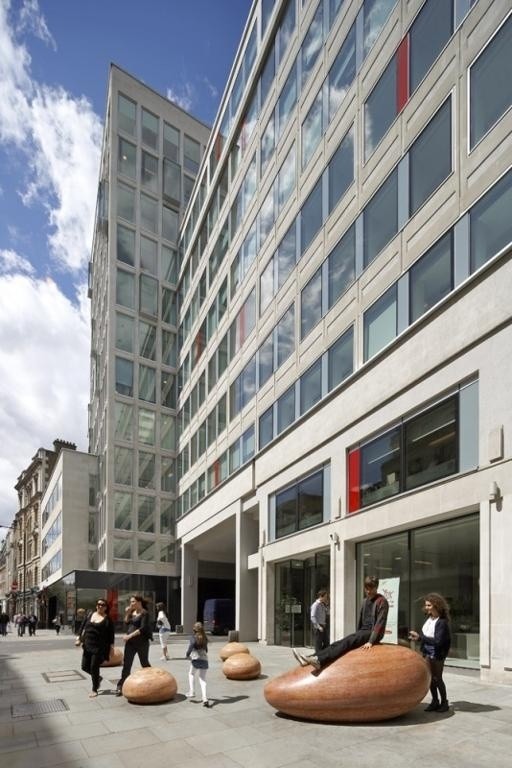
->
left=301, top=655, right=321, bottom=670
left=160, top=653, right=169, bottom=660
left=116, top=689, right=123, bottom=697
left=424, top=700, right=440, bottom=713
left=185, top=693, right=196, bottom=698
left=435, top=699, right=449, bottom=713
left=292, top=648, right=309, bottom=667
left=97, top=676, right=103, bottom=689
left=204, top=701, right=209, bottom=706
left=89, top=691, right=98, bottom=698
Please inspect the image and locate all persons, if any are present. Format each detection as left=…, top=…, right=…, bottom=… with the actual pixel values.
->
left=1, top=607, right=92, bottom=637
left=310, top=588, right=330, bottom=654
left=74, top=598, right=115, bottom=698
left=407, top=592, right=451, bottom=713
left=290, top=574, right=388, bottom=672
left=185, top=622, right=211, bottom=707
left=154, top=602, right=171, bottom=661
left=115, top=595, right=154, bottom=696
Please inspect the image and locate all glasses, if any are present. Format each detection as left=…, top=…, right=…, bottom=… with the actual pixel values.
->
left=98, top=603, right=105, bottom=607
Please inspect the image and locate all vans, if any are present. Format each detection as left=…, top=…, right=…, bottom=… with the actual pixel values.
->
left=203, top=598, right=235, bottom=635
